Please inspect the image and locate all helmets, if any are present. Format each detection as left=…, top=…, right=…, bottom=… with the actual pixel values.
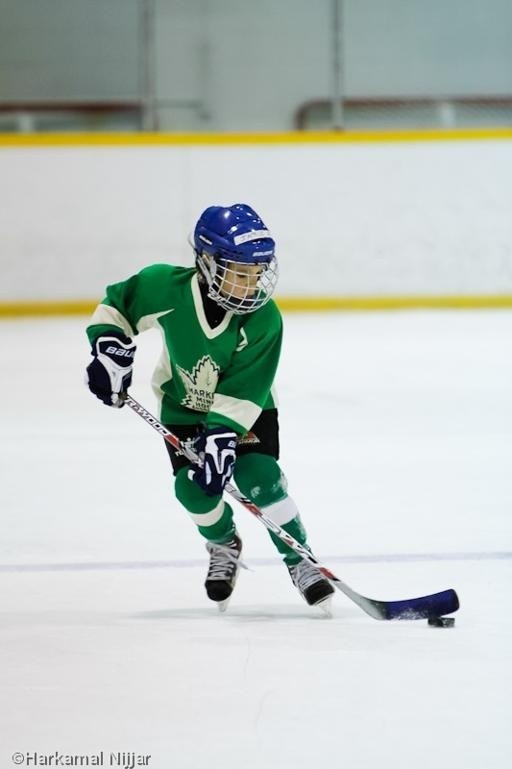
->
left=194, top=204, right=280, bottom=315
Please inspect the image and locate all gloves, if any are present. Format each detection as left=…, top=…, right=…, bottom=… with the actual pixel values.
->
left=86, top=335, right=136, bottom=408
left=187, top=428, right=237, bottom=494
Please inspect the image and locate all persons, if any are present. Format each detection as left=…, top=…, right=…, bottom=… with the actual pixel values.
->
left=83, top=203, right=337, bottom=611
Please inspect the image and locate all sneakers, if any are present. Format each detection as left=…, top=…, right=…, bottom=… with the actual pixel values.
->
left=288, top=558, right=334, bottom=606
left=204, top=536, right=253, bottom=601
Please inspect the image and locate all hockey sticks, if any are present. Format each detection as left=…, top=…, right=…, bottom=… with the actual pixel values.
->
left=123, top=395, right=459, bottom=622
